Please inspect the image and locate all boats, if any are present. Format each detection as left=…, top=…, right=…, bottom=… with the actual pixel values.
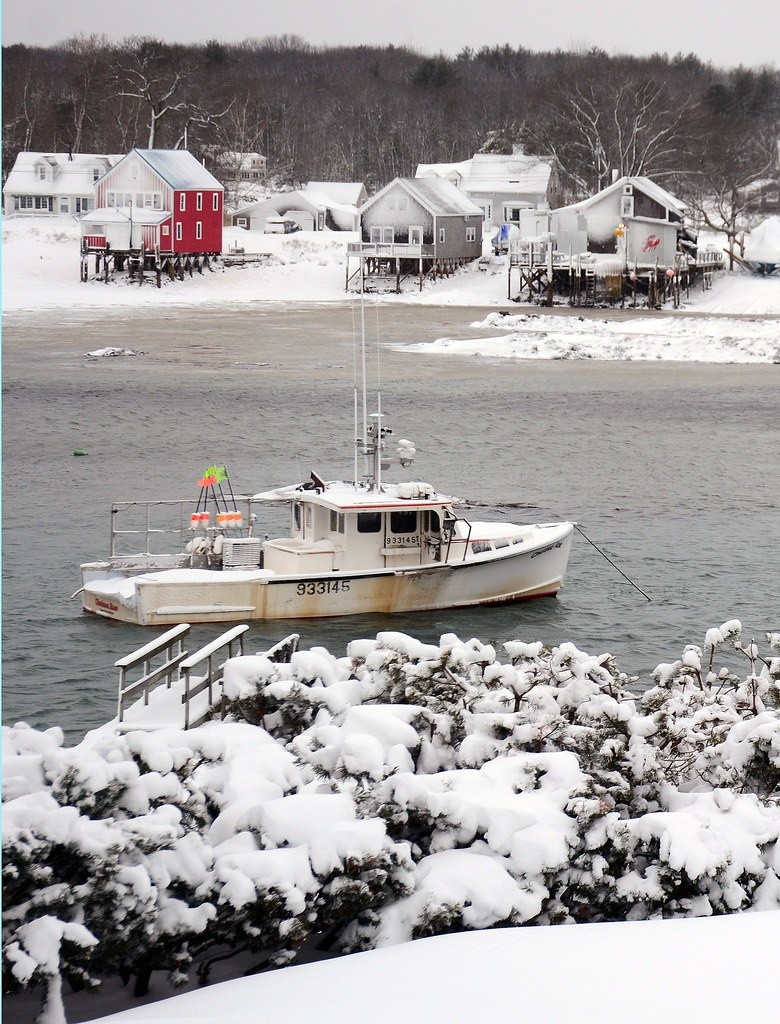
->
left=70, top=224, right=579, bottom=628
